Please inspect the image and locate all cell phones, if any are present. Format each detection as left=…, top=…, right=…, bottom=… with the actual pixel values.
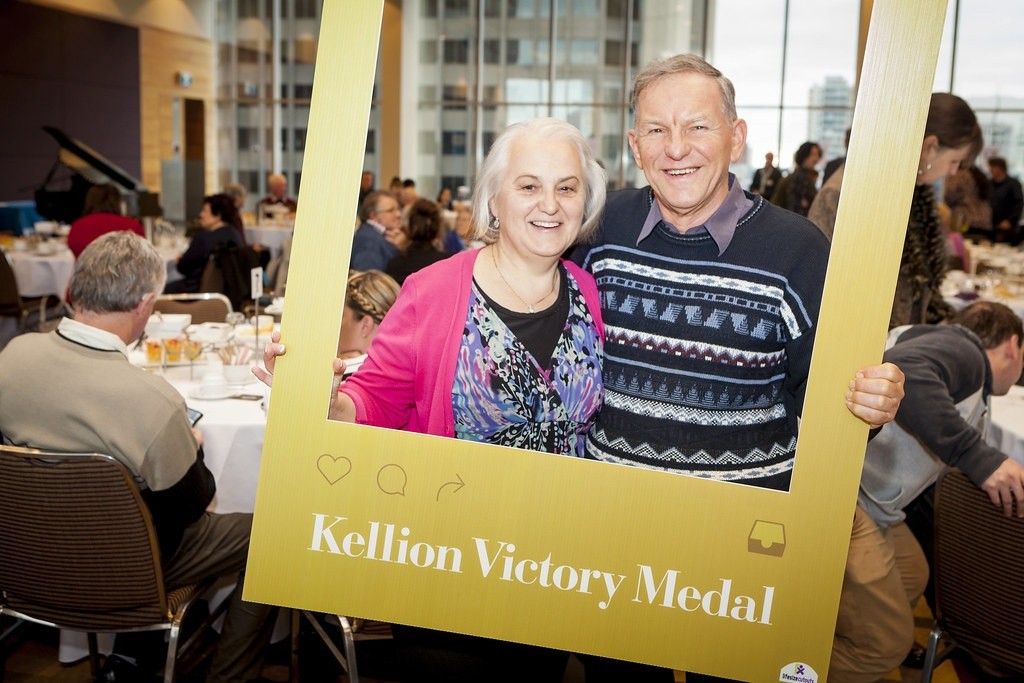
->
left=186, top=408, right=203, bottom=427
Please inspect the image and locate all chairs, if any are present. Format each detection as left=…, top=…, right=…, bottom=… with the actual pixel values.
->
left=0, top=442, right=245, bottom=683
left=286, top=603, right=395, bottom=683
left=148, top=292, right=233, bottom=327
left=922, top=469, right=1024, bottom=683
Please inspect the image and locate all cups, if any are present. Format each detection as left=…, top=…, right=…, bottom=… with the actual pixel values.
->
left=252, top=314, right=273, bottom=343
left=223, top=364, right=251, bottom=381
left=144, top=338, right=203, bottom=361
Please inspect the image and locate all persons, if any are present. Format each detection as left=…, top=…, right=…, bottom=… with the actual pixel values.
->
left=392, top=177, right=461, bottom=211
left=382, top=199, right=468, bottom=288
left=808, top=94, right=985, bottom=333
left=742, top=93, right=1024, bottom=248
left=260, top=174, right=297, bottom=213
left=224, top=182, right=271, bottom=272
left=331, top=270, right=402, bottom=378
left=357, top=173, right=376, bottom=213
left=349, top=190, right=412, bottom=268
left=172, top=194, right=271, bottom=317
left=560, top=53, right=906, bottom=683
left=68, top=184, right=142, bottom=301
left=251, top=116, right=610, bottom=683
left=0, top=229, right=280, bottom=683
left=826, top=302, right=1024, bottom=683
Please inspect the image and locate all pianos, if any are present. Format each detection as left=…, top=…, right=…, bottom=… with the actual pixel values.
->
left=34, top=126, right=166, bottom=225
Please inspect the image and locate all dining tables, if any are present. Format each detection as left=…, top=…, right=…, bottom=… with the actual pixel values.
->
left=139, top=325, right=341, bottom=643
left=941, top=270, right=1024, bottom=322
left=2, top=227, right=189, bottom=330
left=984, top=387, right=1024, bottom=466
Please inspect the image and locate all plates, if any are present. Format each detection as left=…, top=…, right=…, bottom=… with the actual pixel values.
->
left=187, top=383, right=241, bottom=400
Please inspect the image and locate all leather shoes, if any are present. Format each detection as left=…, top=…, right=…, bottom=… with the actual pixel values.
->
left=101, top=654, right=164, bottom=683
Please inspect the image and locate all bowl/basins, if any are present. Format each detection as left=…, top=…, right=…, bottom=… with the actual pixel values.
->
left=144, top=313, right=192, bottom=338
left=194, top=321, right=233, bottom=343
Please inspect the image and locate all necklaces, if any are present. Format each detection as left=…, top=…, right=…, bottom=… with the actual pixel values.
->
left=491, top=242, right=558, bottom=313
